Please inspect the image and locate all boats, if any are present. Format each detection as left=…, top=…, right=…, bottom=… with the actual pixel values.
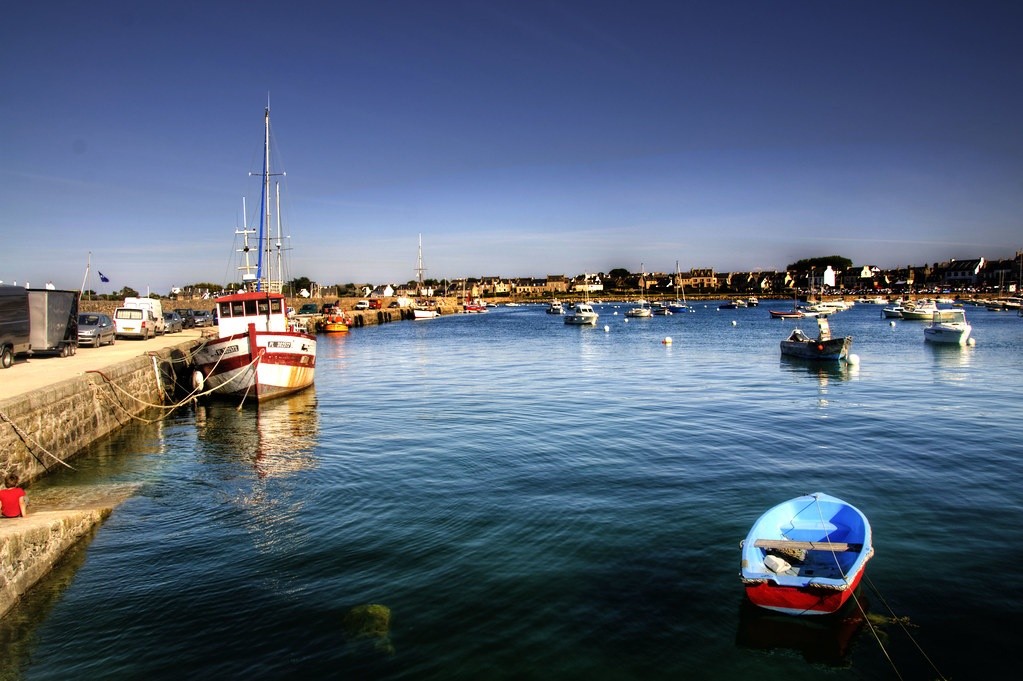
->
left=625, top=308, right=651, bottom=318
left=719, top=297, right=759, bottom=310
left=633, top=261, right=687, bottom=316
left=324, top=306, right=348, bottom=332
left=769, top=298, right=855, bottom=318
left=464, top=302, right=519, bottom=313
left=546, top=270, right=602, bottom=314
left=781, top=326, right=853, bottom=361
left=564, top=304, right=599, bottom=325
left=964, top=297, right=1023, bottom=310
left=742, top=491, right=874, bottom=616
left=924, top=309, right=971, bottom=344
left=853, top=298, right=955, bottom=321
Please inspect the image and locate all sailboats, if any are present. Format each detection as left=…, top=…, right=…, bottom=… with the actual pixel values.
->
left=191, top=91, right=319, bottom=404
left=411, top=232, right=440, bottom=317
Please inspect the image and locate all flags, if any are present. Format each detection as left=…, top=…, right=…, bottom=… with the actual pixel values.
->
left=98, top=270, right=109, bottom=282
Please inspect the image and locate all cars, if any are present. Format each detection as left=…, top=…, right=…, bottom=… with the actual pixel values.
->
left=417, top=300, right=438, bottom=306
left=287, top=298, right=400, bottom=320
left=0, top=284, right=219, bottom=369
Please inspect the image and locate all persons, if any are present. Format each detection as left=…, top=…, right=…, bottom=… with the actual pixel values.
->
left=327, top=313, right=352, bottom=325
